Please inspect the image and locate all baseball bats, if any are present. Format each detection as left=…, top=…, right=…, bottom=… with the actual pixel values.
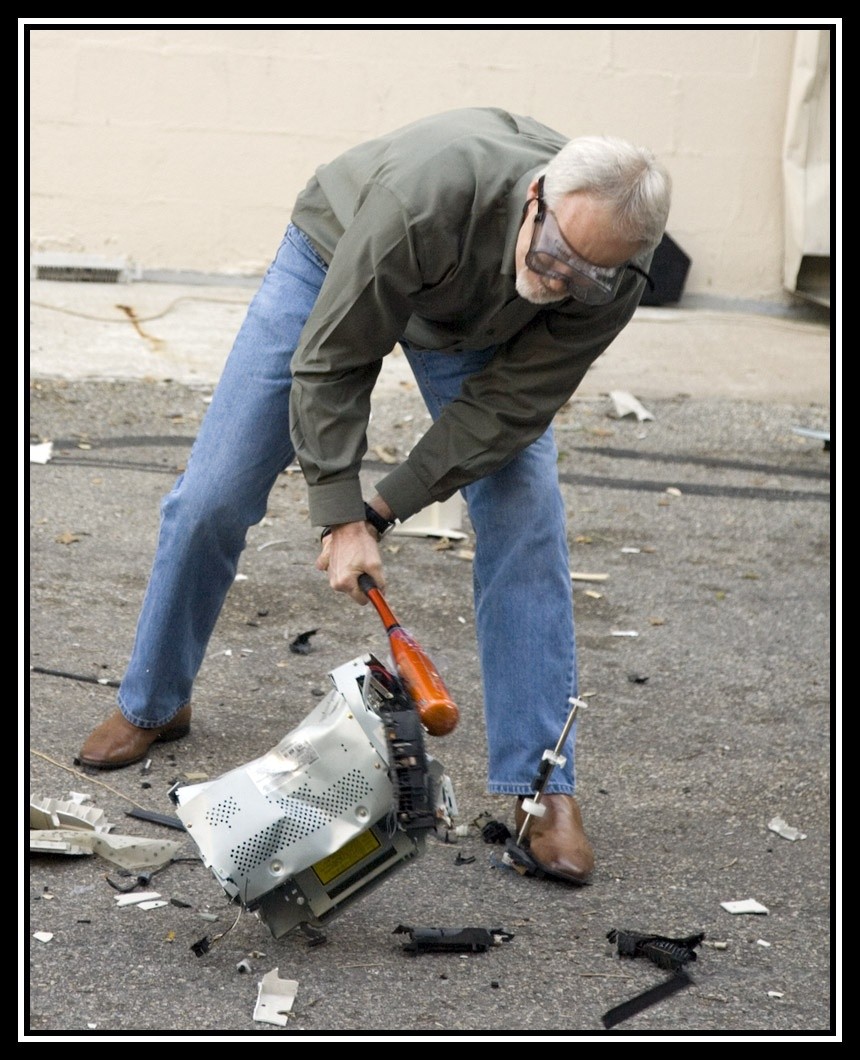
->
left=356, top=571, right=459, bottom=738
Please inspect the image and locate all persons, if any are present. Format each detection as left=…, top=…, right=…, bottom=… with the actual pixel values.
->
left=78, top=106, right=672, bottom=887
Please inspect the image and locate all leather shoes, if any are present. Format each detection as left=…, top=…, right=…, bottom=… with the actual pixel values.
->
left=514, top=792, right=594, bottom=884
left=78, top=701, right=194, bottom=767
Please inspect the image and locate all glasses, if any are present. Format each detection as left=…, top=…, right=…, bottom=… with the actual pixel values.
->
left=524, top=175, right=634, bottom=305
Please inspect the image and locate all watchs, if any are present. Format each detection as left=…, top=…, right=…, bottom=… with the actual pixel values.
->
left=365, top=501, right=395, bottom=543
